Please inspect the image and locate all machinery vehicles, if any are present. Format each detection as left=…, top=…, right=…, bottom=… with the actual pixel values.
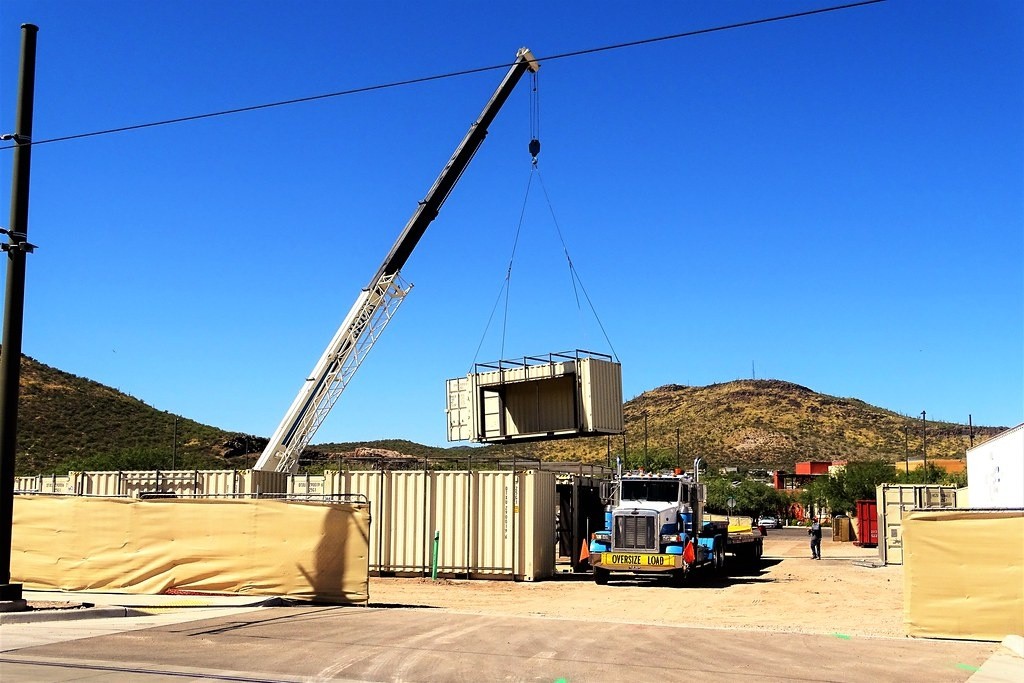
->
left=254, top=50, right=542, bottom=475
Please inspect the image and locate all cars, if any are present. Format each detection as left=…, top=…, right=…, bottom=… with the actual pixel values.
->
left=758, top=516, right=779, bottom=528
left=686, top=466, right=773, bottom=488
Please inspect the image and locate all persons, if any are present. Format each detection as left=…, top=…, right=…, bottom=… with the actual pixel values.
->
left=806, top=517, right=822, bottom=561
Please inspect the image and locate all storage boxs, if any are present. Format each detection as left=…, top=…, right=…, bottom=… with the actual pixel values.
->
left=444, top=358, right=623, bottom=443
left=14, top=474, right=67, bottom=494
left=67, top=469, right=289, bottom=501
left=286, top=475, right=323, bottom=502
left=324, top=469, right=555, bottom=583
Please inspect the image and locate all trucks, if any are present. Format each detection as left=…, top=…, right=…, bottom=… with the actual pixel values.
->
left=588, top=460, right=766, bottom=587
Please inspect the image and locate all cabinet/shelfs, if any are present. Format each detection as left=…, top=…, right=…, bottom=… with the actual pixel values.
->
left=831, top=518, right=850, bottom=542
